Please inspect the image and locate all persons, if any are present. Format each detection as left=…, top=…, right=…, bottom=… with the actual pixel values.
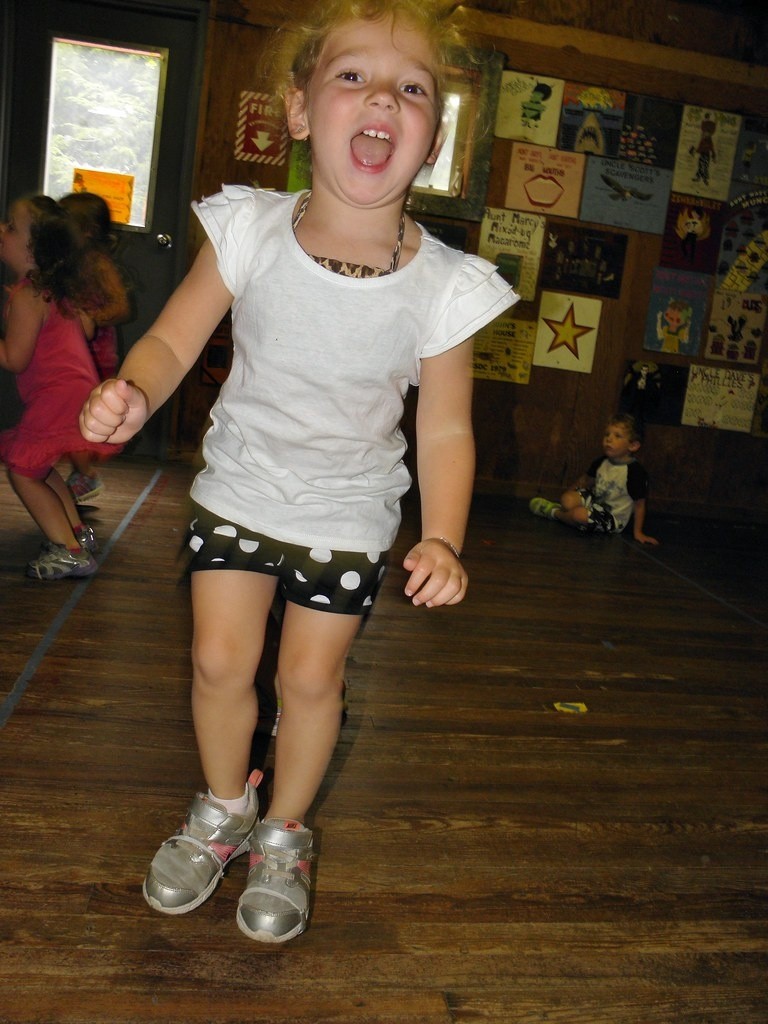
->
left=0, top=193, right=133, bottom=581
left=79, top=0, right=523, bottom=942
left=529, top=414, right=661, bottom=545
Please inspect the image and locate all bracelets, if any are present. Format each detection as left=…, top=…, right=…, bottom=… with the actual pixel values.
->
left=436, top=536, right=459, bottom=562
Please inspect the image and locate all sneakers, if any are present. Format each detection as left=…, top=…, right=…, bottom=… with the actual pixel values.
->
left=530, top=498, right=563, bottom=519
left=26, top=543, right=101, bottom=580
left=142, top=769, right=265, bottom=916
left=236, top=813, right=315, bottom=944
left=42, top=524, right=99, bottom=555
left=66, top=471, right=106, bottom=502
